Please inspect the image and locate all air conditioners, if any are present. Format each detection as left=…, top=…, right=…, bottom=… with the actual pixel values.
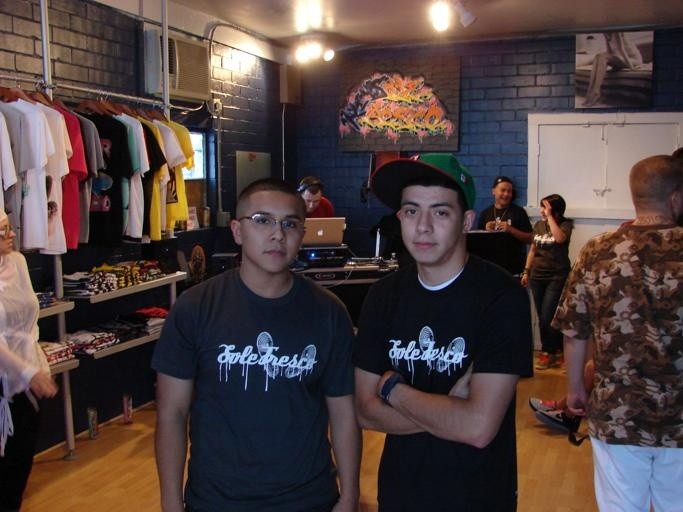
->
left=142, top=29, right=210, bottom=104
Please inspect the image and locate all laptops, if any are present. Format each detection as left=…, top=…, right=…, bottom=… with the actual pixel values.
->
left=301, top=218, right=345, bottom=245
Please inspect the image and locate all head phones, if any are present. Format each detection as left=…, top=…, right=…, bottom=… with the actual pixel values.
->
left=298, top=179, right=324, bottom=193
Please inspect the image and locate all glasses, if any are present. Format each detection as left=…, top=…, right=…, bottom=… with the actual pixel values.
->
left=238, top=212, right=306, bottom=233
left=0, top=224, right=13, bottom=240
left=557, top=411, right=589, bottom=446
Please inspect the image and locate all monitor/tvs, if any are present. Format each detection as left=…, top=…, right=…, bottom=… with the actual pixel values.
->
left=183, top=131, right=207, bottom=181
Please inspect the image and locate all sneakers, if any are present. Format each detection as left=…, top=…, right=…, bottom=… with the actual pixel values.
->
left=536, top=353, right=550, bottom=369
left=528, top=396, right=567, bottom=411
left=552, top=353, right=563, bottom=366
left=536, top=408, right=582, bottom=434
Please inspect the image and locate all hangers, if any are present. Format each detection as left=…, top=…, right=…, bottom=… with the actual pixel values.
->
left=0, top=76, right=168, bottom=122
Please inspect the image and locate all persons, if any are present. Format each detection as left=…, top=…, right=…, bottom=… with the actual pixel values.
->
left=581, top=35, right=643, bottom=106
left=551, top=155, right=683, bottom=512
left=1, top=209, right=58, bottom=511
left=521, top=193, right=573, bottom=371
left=527, top=361, right=596, bottom=432
left=356, top=154, right=535, bottom=511
left=151, top=178, right=362, bottom=512
left=299, top=176, right=334, bottom=217
left=479, top=176, right=534, bottom=245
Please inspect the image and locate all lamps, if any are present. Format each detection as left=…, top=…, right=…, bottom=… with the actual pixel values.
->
left=454, top=2, right=477, bottom=29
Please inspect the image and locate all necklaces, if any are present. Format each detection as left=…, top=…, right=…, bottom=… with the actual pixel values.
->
left=494, top=204, right=508, bottom=230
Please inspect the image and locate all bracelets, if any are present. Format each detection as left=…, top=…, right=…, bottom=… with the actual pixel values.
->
left=523, top=268, right=530, bottom=275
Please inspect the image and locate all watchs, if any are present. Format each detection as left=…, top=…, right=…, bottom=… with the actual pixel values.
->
left=378, top=371, right=406, bottom=401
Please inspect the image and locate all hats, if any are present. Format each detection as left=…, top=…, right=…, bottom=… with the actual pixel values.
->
left=491, top=175, right=512, bottom=189
left=372, top=152, right=476, bottom=209
